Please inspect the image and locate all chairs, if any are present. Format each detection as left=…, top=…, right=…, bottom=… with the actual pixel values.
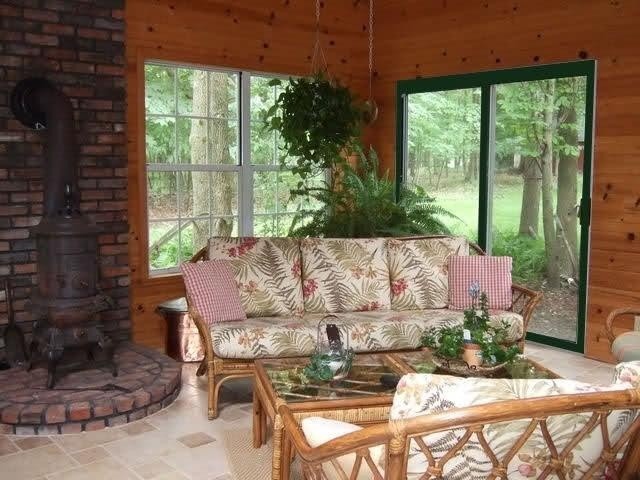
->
left=607, top=307, right=640, bottom=362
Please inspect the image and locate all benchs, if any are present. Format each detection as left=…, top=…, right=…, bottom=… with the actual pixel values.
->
left=277, top=364, right=640, bottom=479
left=183, top=236, right=542, bottom=421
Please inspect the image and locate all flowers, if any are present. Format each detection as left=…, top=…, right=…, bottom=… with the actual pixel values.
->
left=419, top=282, right=519, bottom=372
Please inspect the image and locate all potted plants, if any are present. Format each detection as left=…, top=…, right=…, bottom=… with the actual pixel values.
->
left=262, top=70, right=366, bottom=192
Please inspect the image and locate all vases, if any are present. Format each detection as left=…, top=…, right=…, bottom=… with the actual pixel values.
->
left=461, top=343, right=487, bottom=368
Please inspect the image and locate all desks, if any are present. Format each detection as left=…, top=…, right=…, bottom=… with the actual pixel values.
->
left=247, top=347, right=565, bottom=461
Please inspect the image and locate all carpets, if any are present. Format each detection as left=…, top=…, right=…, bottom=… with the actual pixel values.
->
left=221, top=425, right=310, bottom=480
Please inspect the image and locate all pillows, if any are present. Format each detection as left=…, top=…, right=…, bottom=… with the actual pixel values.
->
left=209, top=235, right=305, bottom=316
left=389, top=372, right=639, bottom=478
left=179, top=260, right=248, bottom=323
left=300, top=238, right=393, bottom=311
left=610, top=363, right=640, bottom=398
left=389, top=238, right=468, bottom=312
left=446, top=254, right=512, bottom=311
